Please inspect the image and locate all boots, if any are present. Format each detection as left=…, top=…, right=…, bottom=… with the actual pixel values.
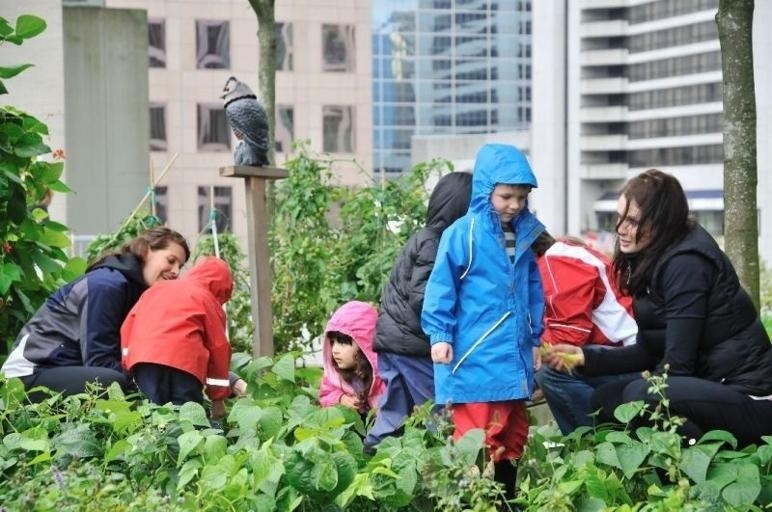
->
left=493, top=458, right=524, bottom=512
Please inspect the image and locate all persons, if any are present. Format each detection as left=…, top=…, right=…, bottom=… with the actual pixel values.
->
left=317, top=300, right=388, bottom=432
left=362, top=171, right=471, bottom=459
left=532, top=229, right=638, bottom=442
left=421, top=143, right=545, bottom=512
left=120, top=256, right=232, bottom=420
left=1, top=228, right=253, bottom=412
left=536, top=168, right=772, bottom=485
